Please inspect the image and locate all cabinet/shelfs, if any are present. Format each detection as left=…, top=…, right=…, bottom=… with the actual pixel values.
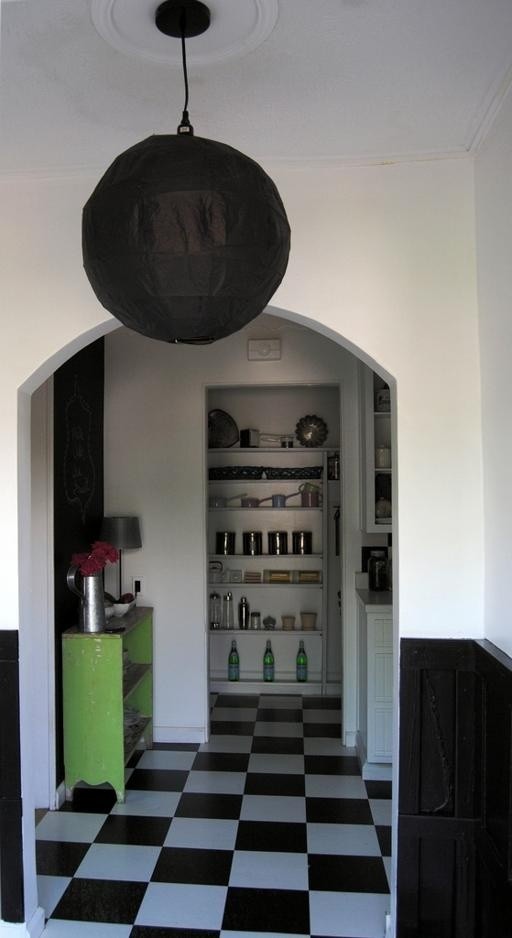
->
left=207, top=448, right=326, bottom=686
left=363, top=371, right=392, bottom=533
left=61, top=608, right=154, bottom=800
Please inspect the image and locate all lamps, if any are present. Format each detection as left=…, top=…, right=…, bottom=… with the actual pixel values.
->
left=81, top=0, right=291, bottom=346
left=99, top=517, right=143, bottom=598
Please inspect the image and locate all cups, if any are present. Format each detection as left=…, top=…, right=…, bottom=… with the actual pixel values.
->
left=302, top=493, right=319, bottom=507
left=281, top=437, right=293, bottom=447
left=251, top=612, right=260, bottom=629
left=299, top=483, right=321, bottom=493
left=375, top=448, right=391, bottom=468
left=210, top=594, right=221, bottom=628
left=223, top=592, right=233, bottom=629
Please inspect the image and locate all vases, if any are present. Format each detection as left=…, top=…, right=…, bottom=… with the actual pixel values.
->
left=66, top=564, right=106, bottom=633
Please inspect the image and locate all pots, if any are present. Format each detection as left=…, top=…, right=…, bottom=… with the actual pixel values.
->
left=240, top=496, right=271, bottom=508
left=209, top=493, right=247, bottom=509
left=272, top=491, right=301, bottom=508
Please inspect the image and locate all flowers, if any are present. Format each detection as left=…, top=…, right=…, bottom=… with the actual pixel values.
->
left=71, top=542, right=120, bottom=578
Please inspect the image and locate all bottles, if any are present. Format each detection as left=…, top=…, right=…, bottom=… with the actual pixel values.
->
left=368, top=551, right=387, bottom=591
left=239, top=596, right=249, bottom=628
left=263, top=640, right=274, bottom=681
left=296, top=640, right=308, bottom=681
left=229, top=640, right=239, bottom=680
